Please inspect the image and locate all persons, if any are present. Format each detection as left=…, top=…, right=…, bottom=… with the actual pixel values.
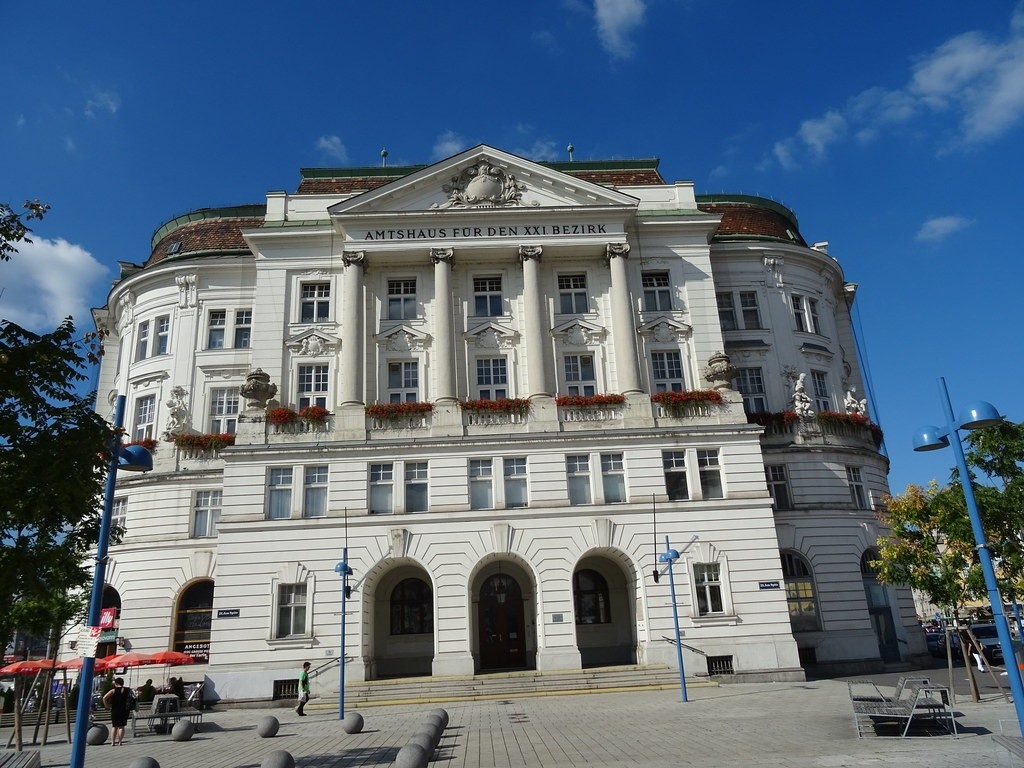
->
left=968, top=636, right=985, bottom=672
left=298, top=662, right=311, bottom=717
left=104, top=678, right=134, bottom=745
left=162, top=399, right=186, bottom=441
left=787, top=373, right=868, bottom=421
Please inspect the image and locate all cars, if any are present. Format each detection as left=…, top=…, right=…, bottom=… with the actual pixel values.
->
left=919, top=602, right=1024, bottom=666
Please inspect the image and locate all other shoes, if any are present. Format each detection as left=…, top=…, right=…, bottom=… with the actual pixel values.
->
left=299, top=713, right=307, bottom=716
left=296, top=710, right=299, bottom=714
left=111, top=744, right=115, bottom=746
left=119, top=743, right=122, bottom=746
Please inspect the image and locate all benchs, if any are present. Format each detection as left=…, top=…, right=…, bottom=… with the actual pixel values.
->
left=0, top=749, right=41, bottom=768
left=848, top=677, right=958, bottom=740
left=131, top=681, right=202, bottom=737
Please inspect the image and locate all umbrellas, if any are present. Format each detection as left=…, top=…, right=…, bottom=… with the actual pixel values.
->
left=0, top=651, right=194, bottom=699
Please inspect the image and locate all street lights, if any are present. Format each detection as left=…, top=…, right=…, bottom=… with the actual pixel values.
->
left=912, top=377, right=1024, bottom=741
left=659, top=536, right=687, bottom=703
left=334, top=548, right=353, bottom=720
left=69, top=395, right=152, bottom=768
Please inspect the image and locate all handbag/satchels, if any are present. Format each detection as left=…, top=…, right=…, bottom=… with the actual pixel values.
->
left=126, top=687, right=136, bottom=711
left=298, top=690, right=306, bottom=702
